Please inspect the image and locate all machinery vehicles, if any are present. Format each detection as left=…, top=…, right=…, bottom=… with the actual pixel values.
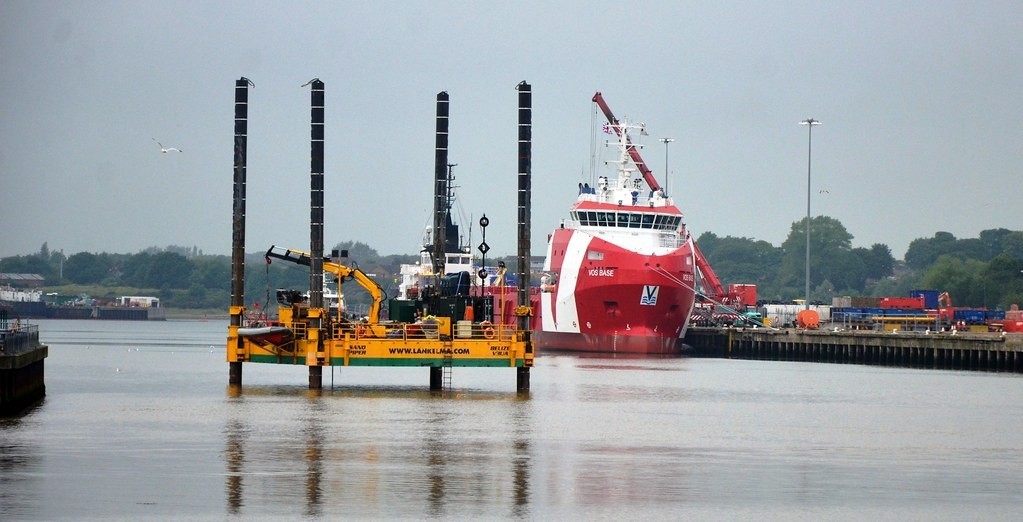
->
left=591, top=90, right=746, bottom=323
left=264, top=243, right=387, bottom=338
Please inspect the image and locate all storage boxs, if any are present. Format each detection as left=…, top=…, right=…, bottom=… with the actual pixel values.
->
left=829, top=290, right=1005, bottom=326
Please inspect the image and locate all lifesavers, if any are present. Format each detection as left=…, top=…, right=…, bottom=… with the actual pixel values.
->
left=483, top=327, right=496, bottom=339
left=480, top=320, right=492, bottom=331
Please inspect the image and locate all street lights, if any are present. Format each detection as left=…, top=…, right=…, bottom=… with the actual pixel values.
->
left=659, top=137, right=675, bottom=196
left=797, top=118, right=823, bottom=310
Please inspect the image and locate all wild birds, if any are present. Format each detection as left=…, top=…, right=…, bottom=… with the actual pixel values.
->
left=820, top=190, right=829, bottom=194
left=152, top=137, right=182, bottom=153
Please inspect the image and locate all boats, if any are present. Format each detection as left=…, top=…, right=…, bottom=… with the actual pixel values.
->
left=388, top=116, right=695, bottom=354
